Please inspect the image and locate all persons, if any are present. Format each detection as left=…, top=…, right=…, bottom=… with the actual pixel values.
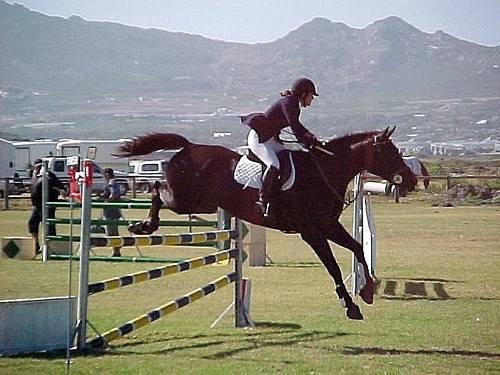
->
left=99, top=168, right=122, bottom=257
left=27, top=159, right=67, bottom=256
left=240, top=78, right=319, bottom=222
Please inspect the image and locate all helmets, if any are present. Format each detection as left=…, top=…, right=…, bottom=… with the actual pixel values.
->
left=291, top=77, right=319, bottom=97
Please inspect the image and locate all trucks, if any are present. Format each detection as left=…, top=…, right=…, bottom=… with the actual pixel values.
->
left=0, top=136, right=182, bottom=198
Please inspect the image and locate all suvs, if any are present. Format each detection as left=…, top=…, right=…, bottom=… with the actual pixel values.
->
left=41, top=153, right=133, bottom=198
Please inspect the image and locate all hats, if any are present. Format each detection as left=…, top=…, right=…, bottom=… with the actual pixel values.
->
left=34, top=159, right=42, bottom=167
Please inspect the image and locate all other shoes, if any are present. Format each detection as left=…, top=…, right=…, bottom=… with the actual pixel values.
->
left=110, top=253, right=121, bottom=257
left=36, top=246, right=42, bottom=254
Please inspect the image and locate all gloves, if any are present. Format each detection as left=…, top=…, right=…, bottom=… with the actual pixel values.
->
left=311, top=139, right=322, bottom=148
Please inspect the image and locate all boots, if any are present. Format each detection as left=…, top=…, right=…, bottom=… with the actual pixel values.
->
left=259, top=164, right=279, bottom=208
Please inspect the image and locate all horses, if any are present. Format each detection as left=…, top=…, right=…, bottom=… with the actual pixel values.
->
left=108, top=124, right=416, bottom=321
left=402, top=156, right=430, bottom=190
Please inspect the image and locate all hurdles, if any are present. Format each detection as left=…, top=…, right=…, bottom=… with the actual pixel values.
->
left=348, top=171, right=397, bottom=300
left=76, top=160, right=251, bottom=355
left=35, top=160, right=232, bottom=264
